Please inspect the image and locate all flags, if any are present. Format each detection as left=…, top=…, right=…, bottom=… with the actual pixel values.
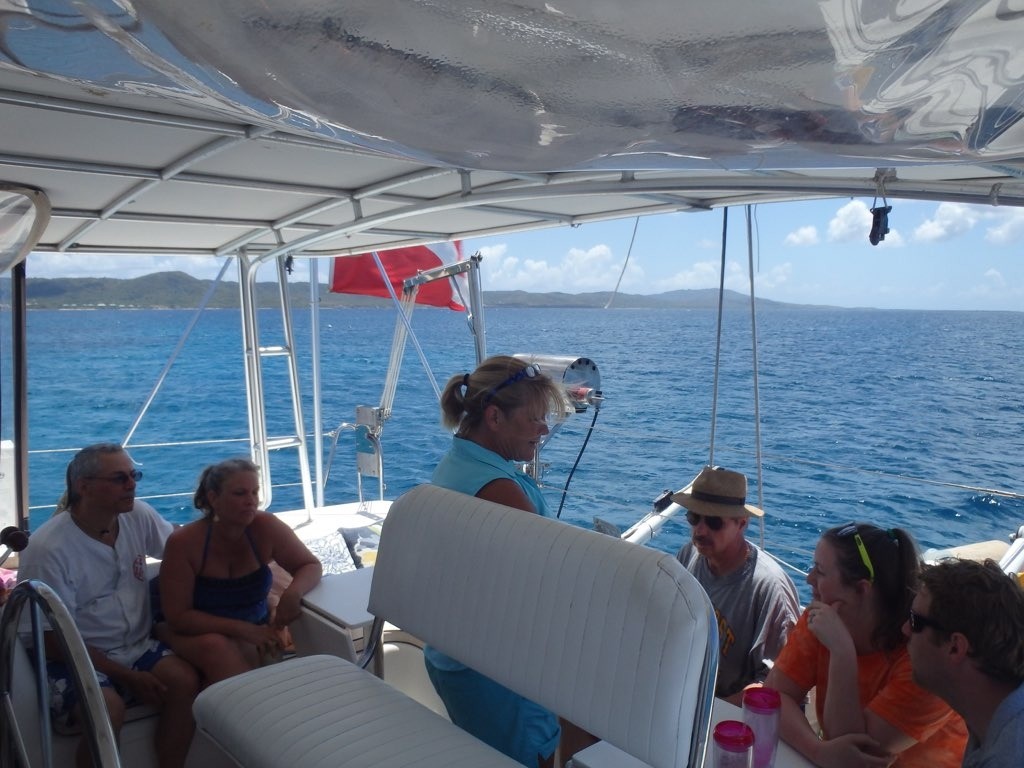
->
left=329, top=240, right=468, bottom=312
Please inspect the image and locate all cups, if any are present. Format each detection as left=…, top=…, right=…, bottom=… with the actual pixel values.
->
left=712, top=719, right=755, bottom=768
left=742, top=687, right=782, bottom=768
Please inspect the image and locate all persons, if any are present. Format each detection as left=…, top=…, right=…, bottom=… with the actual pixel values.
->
left=17, top=444, right=203, bottom=767
left=424, top=358, right=588, bottom=768
left=151, top=458, right=322, bottom=684
left=763, top=520, right=1024, bottom=768
left=671, top=466, right=810, bottom=717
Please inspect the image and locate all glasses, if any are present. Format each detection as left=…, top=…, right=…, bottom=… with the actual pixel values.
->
left=686, top=510, right=739, bottom=529
left=82, top=471, right=143, bottom=483
left=837, top=520, right=876, bottom=586
left=908, top=611, right=975, bottom=659
left=485, top=365, right=541, bottom=403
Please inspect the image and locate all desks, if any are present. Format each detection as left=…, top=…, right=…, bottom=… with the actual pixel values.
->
left=283, top=565, right=375, bottom=666
left=565, top=684, right=823, bottom=768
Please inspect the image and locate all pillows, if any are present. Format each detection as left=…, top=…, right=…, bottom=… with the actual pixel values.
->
left=336, top=521, right=385, bottom=568
left=304, top=532, right=357, bottom=575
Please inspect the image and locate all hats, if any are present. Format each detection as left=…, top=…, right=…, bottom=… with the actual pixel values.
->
left=670, top=465, right=765, bottom=519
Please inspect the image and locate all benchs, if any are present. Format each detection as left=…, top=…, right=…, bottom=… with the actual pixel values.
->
left=0, top=518, right=389, bottom=768
left=180, top=483, right=717, bottom=768
